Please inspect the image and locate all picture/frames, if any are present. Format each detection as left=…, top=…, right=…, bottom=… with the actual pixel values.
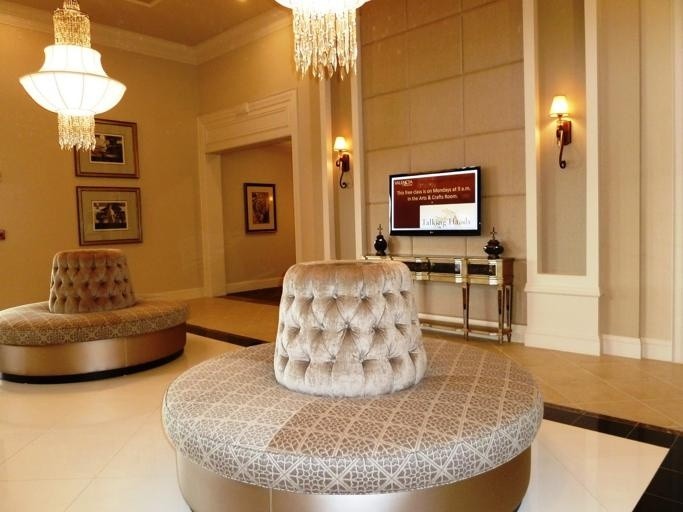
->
left=242, top=182, right=277, bottom=234
left=72, top=116, right=143, bottom=247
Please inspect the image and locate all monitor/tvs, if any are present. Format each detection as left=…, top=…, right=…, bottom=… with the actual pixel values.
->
left=389, top=165, right=481, bottom=236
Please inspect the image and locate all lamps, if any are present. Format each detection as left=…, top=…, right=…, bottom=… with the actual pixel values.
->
left=273, top=1, right=369, bottom=83
left=332, top=135, right=350, bottom=189
left=547, top=94, right=574, bottom=171
left=17, top=1, right=128, bottom=154
